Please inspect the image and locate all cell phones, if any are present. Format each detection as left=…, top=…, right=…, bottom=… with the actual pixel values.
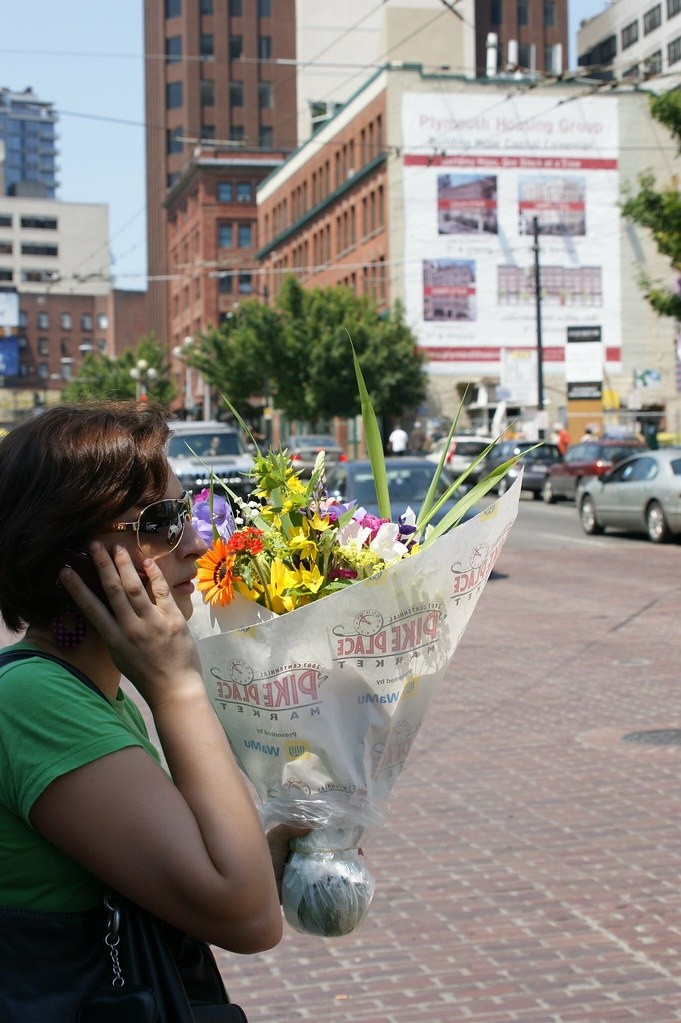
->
left=45, top=547, right=149, bottom=618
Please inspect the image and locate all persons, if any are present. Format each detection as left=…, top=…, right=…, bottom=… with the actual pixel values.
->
left=388, top=424, right=409, bottom=456
left=580, top=429, right=598, bottom=444
left=0, top=400, right=284, bottom=1023
left=554, top=424, right=570, bottom=455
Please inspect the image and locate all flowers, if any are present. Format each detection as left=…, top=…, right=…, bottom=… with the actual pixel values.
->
left=179, top=326, right=547, bottom=940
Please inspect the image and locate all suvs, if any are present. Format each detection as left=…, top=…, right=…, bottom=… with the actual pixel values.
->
left=168, top=425, right=264, bottom=508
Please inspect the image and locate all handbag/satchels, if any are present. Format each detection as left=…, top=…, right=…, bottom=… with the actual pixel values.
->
left=1, top=647, right=245, bottom=1022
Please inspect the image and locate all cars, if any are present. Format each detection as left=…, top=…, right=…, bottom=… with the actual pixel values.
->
left=479, top=442, right=563, bottom=499
left=326, top=457, right=481, bottom=545
left=425, top=436, right=500, bottom=488
left=574, top=448, right=681, bottom=543
left=542, top=440, right=652, bottom=505
left=280, top=436, right=346, bottom=483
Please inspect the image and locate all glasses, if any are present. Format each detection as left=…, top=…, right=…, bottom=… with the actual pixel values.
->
left=103, top=491, right=194, bottom=557
